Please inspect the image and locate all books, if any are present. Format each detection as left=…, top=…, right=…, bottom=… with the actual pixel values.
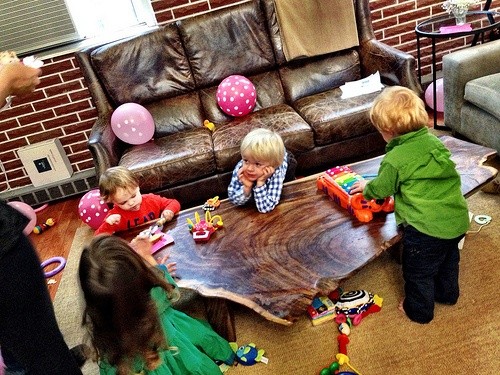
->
left=307, top=296, right=337, bottom=325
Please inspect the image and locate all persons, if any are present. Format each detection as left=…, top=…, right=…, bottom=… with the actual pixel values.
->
left=349, top=86, right=470, bottom=324
left=0, top=63, right=91, bottom=375
left=94, top=166, right=180, bottom=233
left=228, top=128, right=297, bottom=213
left=78, top=234, right=236, bottom=375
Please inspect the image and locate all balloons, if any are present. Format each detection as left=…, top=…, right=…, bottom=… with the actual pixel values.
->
left=79, top=190, right=110, bottom=229
left=425, top=78, right=444, bottom=112
left=110, top=103, right=155, bottom=144
left=217, top=75, right=257, bottom=116
left=7, top=201, right=36, bottom=235
